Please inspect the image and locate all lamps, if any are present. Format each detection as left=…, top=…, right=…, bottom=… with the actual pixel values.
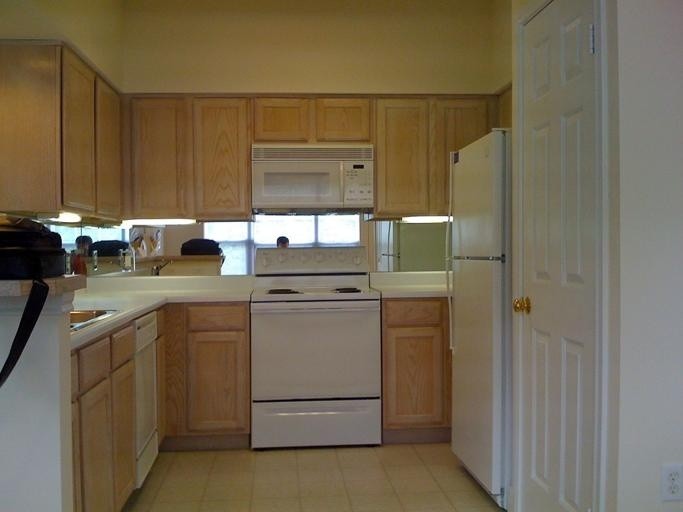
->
left=399, top=216, right=453, bottom=223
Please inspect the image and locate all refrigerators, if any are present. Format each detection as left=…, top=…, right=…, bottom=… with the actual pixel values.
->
left=134, top=311, right=158, bottom=489
left=393, top=220, right=451, bottom=272
left=445, top=128, right=510, bottom=508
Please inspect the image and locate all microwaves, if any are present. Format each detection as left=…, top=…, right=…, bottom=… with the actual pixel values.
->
left=251, top=144, right=373, bottom=215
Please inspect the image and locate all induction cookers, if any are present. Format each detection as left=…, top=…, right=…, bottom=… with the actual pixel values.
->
left=250, top=287, right=380, bottom=302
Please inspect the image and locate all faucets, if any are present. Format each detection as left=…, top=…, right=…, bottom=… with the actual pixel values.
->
left=150, top=257, right=173, bottom=275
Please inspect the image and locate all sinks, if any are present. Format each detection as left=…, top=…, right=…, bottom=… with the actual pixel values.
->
left=86, top=270, right=254, bottom=290
left=69, top=309, right=120, bottom=333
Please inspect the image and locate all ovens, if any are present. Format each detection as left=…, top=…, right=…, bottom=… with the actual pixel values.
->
left=250, top=303, right=383, bottom=447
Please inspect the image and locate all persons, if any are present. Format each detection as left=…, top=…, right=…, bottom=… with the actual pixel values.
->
left=69, top=235, right=92, bottom=275
left=276, top=236, right=289, bottom=248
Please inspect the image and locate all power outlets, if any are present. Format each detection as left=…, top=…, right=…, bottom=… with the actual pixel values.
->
left=659, top=463, right=683, bottom=500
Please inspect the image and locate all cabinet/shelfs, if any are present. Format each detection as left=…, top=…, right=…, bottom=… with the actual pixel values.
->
left=156, top=302, right=251, bottom=451
left=374, top=87, right=512, bottom=222
left=253, top=96, right=371, bottom=141
left=381, top=297, right=453, bottom=444
left=72, top=324, right=135, bottom=512
left=0, top=38, right=124, bottom=226
left=125, top=92, right=252, bottom=219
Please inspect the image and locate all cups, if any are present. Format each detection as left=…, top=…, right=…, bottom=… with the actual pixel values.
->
left=145, top=226, right=163, bottom=256
left=127, top=226, right=145, bottom=257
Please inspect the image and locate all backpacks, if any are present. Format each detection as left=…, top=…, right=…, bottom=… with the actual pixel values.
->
left=181, top=238, right=224, bottom=256
left=89, top=240, right=130, bottom=257
left=1, top=217, right=68, bottom=280
